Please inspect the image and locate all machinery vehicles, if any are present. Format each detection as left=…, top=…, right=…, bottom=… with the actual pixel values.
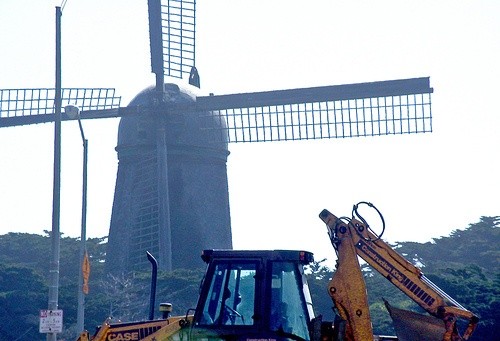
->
left=74, top=202, right=478, bottom=341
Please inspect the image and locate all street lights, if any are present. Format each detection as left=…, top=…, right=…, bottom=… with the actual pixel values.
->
left=65, top=104, right=90, bottom=341
